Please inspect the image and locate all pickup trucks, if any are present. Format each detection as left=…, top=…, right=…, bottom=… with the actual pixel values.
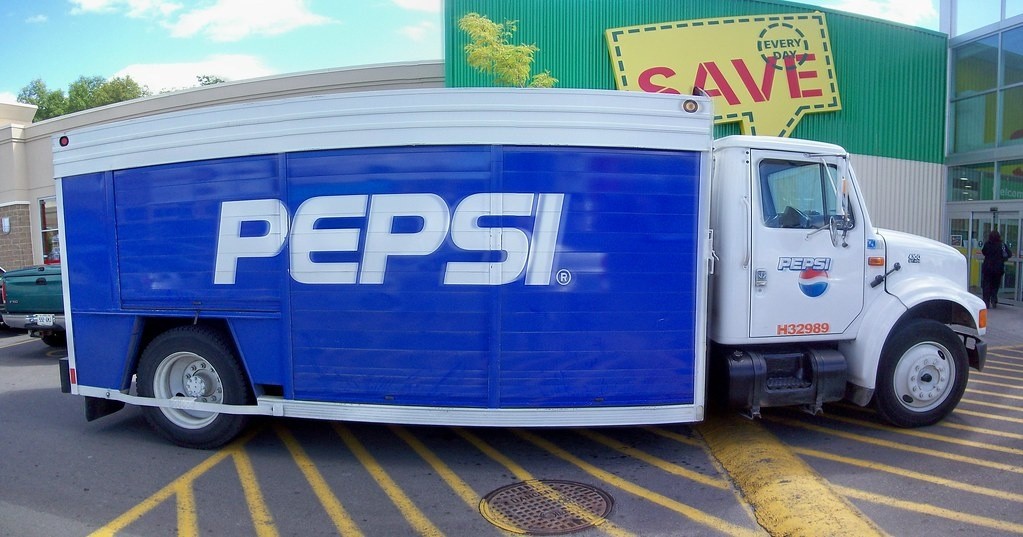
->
left=0, top=262, right=67, bottom=347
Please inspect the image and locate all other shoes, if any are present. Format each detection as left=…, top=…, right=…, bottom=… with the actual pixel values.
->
left=992, top=303, right=997, bottom=308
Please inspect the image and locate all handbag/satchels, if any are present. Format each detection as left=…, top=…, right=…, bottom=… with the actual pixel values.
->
left=1001, top=242, right=1009, bottom=262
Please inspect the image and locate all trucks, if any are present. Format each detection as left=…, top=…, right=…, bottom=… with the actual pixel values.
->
left=49, top=87, right=991, bottom=455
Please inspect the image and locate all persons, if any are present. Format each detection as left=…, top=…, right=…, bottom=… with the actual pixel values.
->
left=981, top=231, right=1012, bottom=309
